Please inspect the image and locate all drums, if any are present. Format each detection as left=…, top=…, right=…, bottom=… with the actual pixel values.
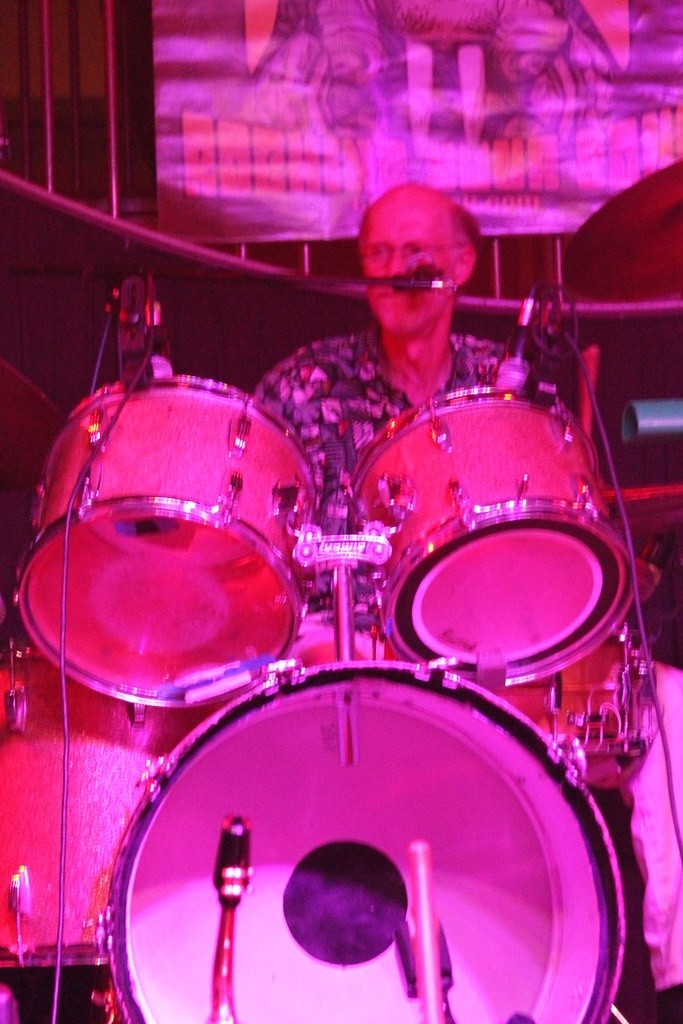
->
left=14, top=374, right=319, bottom=710
left=488, top=631, right=643, bottom=784
left=4, top=644, right=291, bottom=975
left=342, top=385, right=631, bottom=692
left=87, top=656, right=629, bottom=1024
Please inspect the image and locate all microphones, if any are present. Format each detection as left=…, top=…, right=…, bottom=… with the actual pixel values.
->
left=144, top=289, right=172, bottom=380
left=405, top=252, right=440, bottom=290
left=497, top=289, right=539, bottom=389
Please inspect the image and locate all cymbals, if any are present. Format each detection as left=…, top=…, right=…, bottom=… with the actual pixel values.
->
left=563, top=155, right=683, bottom=301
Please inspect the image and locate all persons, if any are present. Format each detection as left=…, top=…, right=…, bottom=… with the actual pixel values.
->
left=255, top=183, right=683, bottom=1023
